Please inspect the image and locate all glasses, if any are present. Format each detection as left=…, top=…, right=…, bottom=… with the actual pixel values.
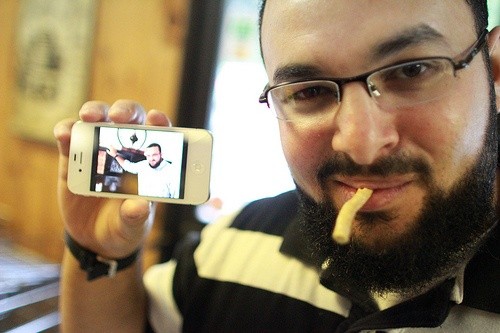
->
left=259, top=28, right=489, bottom=123
left=145, top=152, right=161, bottom=158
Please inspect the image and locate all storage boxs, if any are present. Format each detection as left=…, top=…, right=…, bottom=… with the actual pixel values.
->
left=123, top=150, right=145, bottom=164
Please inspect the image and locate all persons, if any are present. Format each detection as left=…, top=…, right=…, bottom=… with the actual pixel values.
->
left=106, top=142, right=180, bottom=199
left=53, top=1, right=500, bottom=333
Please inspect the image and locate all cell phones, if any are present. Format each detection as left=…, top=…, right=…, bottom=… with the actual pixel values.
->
left=67, top=121, right=214, bottom=206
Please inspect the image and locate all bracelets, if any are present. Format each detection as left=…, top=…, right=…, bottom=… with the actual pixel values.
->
left=61, top=224, right=143, bottom=282
